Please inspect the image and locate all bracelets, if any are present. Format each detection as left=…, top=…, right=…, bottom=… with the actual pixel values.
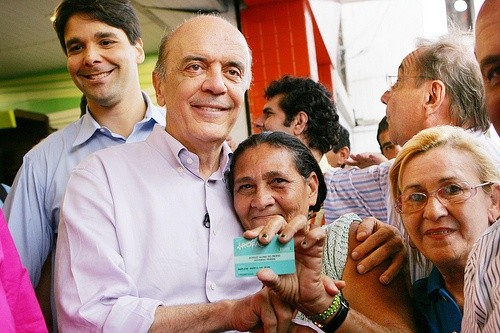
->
left=305, top=289, right=340, bottom=322
left=312, top=297, right=350, bottom=333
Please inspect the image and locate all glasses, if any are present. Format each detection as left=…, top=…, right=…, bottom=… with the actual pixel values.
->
left=384, top=71, right=433, bottom=91
left=395, top=181, right=492, bottom=214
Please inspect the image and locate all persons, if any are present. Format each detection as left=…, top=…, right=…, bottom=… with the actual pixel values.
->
left=1, top=0, right=166, bottom=333
left=253, top=0, right=500, bottom=333
left=227, top=131, right=414, bottom=333
left=54, top=10, right=408, bottom=333
